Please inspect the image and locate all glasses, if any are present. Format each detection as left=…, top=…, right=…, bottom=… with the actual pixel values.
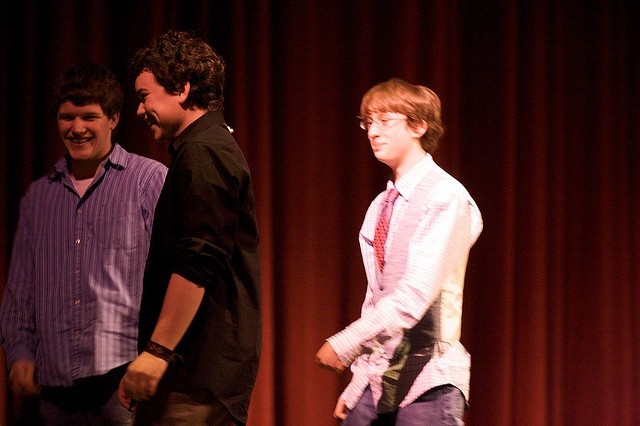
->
left=359, top=115, right=407, bottom=130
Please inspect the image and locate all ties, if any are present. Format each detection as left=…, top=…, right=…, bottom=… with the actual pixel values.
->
left=374, top=188, right=400, bottom=271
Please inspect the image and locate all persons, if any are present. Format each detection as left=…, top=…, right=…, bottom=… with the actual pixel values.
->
left=2, top=67, right=167, bottom=423
left=118, top=34, right=263, bottom=425
left=316, top=79, right=486, bottom=424
